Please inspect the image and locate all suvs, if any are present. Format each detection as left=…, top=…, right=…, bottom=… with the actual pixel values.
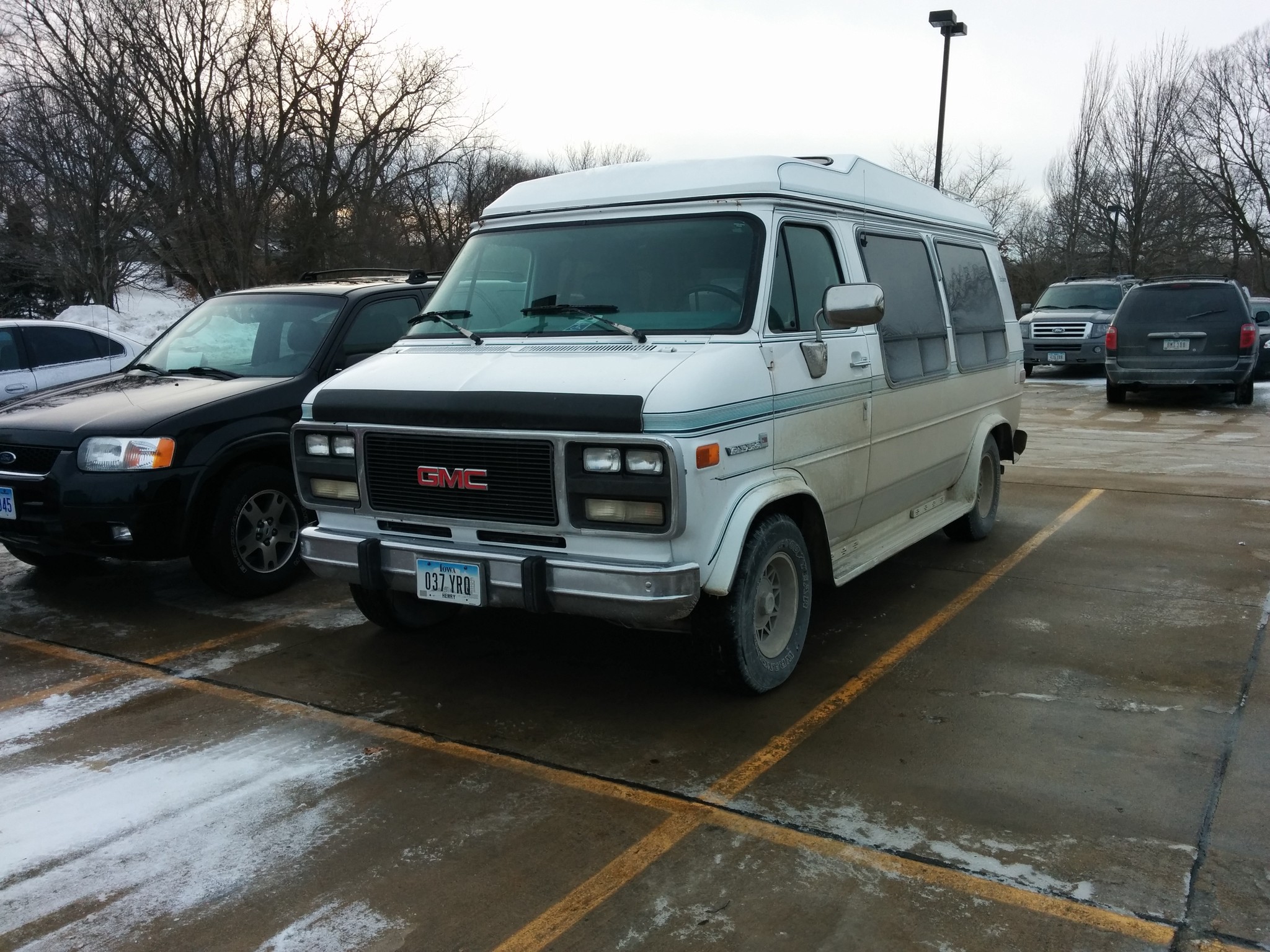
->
left=1106, top=274, right=1261, bottom=405
left=1019, top=274, right=1144, bottom=377
left=0, top=269, right=531, bottom=583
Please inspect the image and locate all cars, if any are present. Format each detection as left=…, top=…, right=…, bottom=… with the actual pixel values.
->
left=1245, top=297, right=1270, bottom=379
left=0, top=320, right=149, bottom=404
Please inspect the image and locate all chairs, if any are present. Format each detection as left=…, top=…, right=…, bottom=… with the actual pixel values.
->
left=688, top=265, right=748, bottom=315
left=271, top=317, right=323, bottom=378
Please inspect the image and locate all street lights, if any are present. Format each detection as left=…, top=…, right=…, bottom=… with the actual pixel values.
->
left=928, top=8, right=968, bottom=191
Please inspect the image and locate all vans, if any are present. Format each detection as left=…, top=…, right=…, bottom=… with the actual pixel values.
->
left=302, top=155, right=1029, bottom=696
